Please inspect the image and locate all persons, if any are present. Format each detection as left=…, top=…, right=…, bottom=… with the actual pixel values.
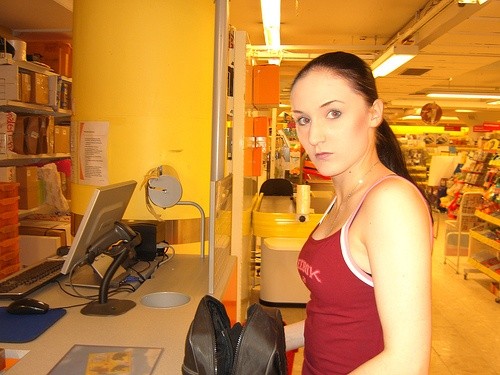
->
left=282, top=49, right=433, bottom=375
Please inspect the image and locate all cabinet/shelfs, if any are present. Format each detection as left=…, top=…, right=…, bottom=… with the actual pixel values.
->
left=0, top=58, right=72, bottom=284
left=444, top=164, right=500, bottom=303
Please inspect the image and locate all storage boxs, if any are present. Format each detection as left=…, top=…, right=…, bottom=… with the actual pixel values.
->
left=53, top=125, right=71, bottom=153
left=0, top=63, right=71, bottom=109
left=0, top=166, right=71, bottom=279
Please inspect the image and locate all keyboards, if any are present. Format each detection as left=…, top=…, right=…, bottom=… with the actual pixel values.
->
left=0, top=258, right=66, bottom=299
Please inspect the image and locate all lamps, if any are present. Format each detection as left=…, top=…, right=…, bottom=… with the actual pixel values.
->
left=370, top=43, right=419, bottom=78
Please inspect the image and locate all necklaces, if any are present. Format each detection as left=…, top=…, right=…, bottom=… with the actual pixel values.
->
left=330, top=159, right=382, bottom=231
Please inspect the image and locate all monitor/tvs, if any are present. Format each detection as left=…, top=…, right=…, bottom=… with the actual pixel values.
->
left=60, top=180, right=137, bottom=275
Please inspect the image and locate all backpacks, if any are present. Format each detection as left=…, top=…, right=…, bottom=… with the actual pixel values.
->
left=182, top=295, right=286, bottom=375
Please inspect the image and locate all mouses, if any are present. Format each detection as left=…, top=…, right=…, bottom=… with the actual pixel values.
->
left=7, top=298, right=49, bottom=314
